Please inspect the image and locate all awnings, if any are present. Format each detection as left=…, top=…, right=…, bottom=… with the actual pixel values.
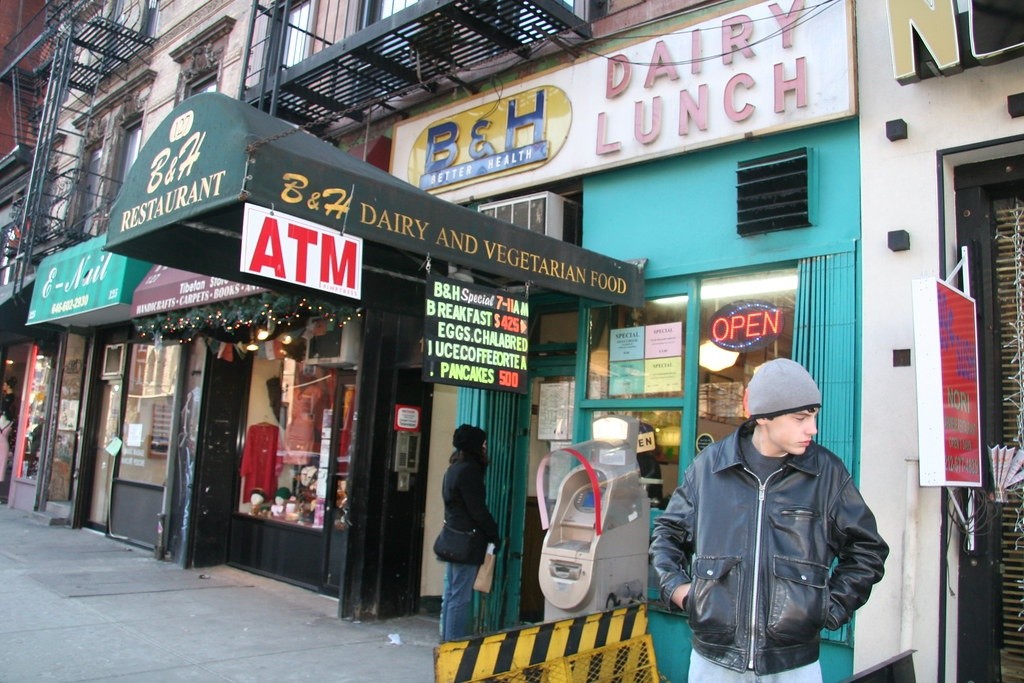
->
left=105, top=92, right=645, bottom=317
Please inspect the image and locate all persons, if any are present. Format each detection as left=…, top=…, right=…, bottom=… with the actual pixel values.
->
left=0, top=376, right=17, bottom=456
left=648, top=357, right=889, bottom=683
left=433, top=423, right=501, bottom=644
left=0, top=414, right=13, bottom=506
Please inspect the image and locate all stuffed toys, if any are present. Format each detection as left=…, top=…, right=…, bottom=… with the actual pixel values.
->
left=250, top=465, right=346, bottom=527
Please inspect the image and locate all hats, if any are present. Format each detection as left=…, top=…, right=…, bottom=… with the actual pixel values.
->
left=452, top=424, right=486, bottom=449
left=746, top=358, right=822, bottom=419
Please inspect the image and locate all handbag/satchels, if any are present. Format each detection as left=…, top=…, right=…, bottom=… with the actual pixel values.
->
left=473, top=552, right=496, bottom=594
